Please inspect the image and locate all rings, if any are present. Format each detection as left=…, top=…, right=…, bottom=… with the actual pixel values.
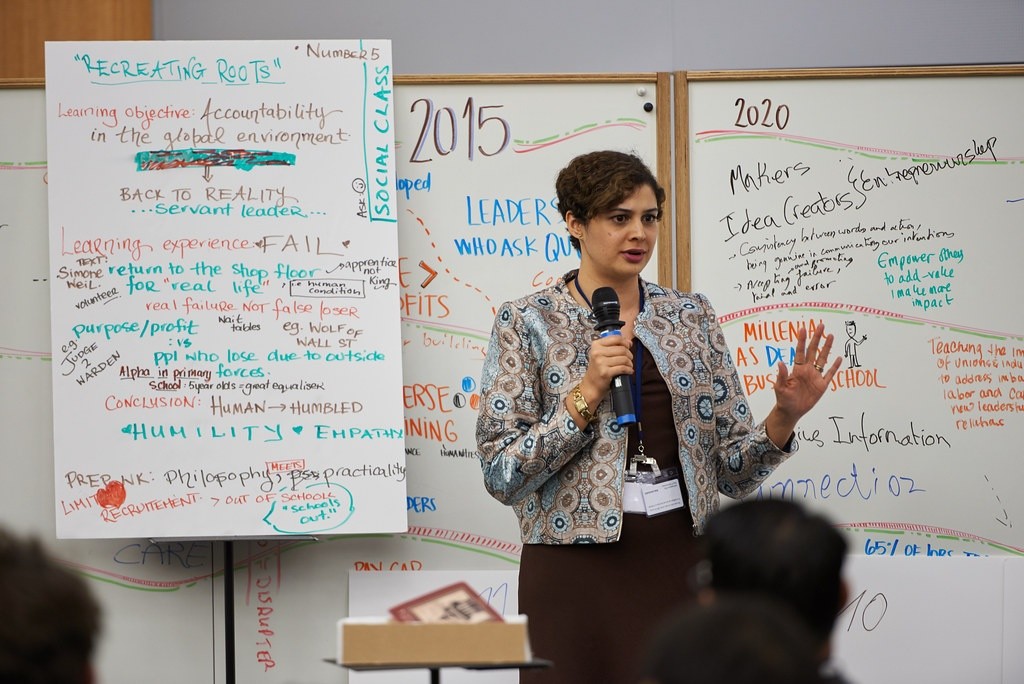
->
left=794, top=360, right=805, bottom=366
left=815, top=365, right=823, bottom=372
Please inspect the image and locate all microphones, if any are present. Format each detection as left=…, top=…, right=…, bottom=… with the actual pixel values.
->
left=592, top=286, right=636, bottom=427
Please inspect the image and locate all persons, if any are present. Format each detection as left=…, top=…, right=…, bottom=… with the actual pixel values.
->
left=474, top=150, right=848, bottom=684
left=0, top=522, right=105, bottom=684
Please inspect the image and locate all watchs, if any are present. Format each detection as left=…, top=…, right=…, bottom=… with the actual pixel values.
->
left=572, top=385, right=599, bottom=423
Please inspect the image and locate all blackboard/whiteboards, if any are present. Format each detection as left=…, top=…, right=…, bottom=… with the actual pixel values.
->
left=674, top=70, right=1023, bottom=555
left=4, top=74, right=671, bottom=684
left=45, top=34, right=409, bottom=541
